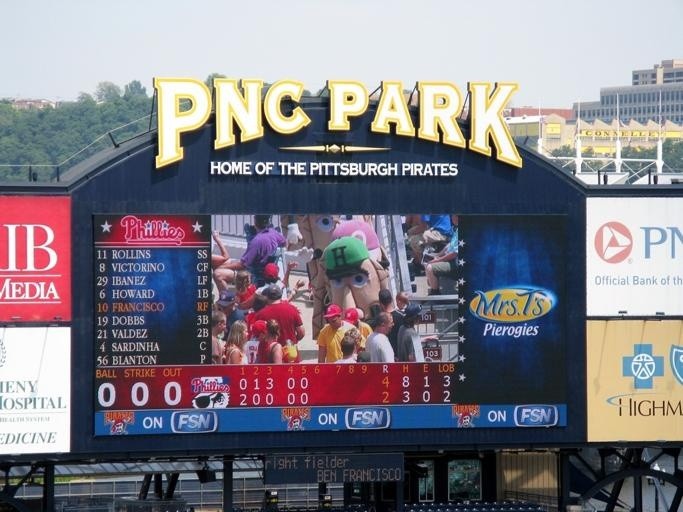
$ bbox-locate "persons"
[210,213,459,364]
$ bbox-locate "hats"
[405,304,422,319]
[216,289,236,307]
[323,304,342,319]
[342,308,358,324]
[264,262,279,278]
[261,285,281,300]
[251,320,267,337]
